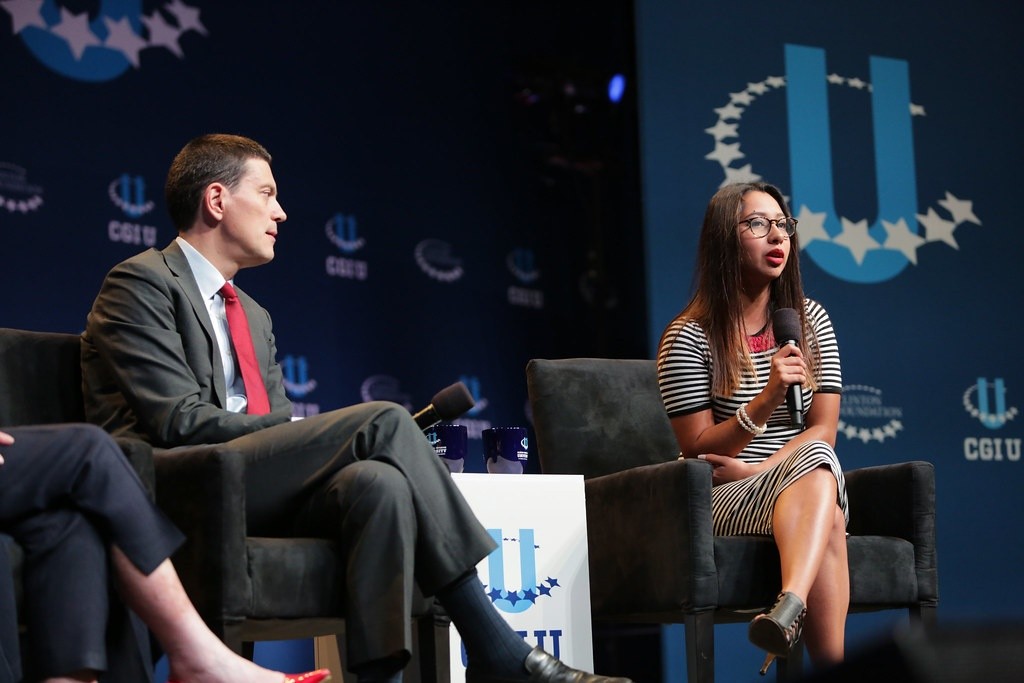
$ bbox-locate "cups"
[424,425,468,473]
[482,426,528,474]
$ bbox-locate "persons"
[655,182,851,668]
[0,423,333,683]
[83,135,632,683]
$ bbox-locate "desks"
[400,471,596,683]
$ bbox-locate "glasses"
[739,217,798,238]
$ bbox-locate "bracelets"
[737,403,767,435]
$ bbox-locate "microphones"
[413,381,475,433]
[771,308,804,426]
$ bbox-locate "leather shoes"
[462,645,633,683]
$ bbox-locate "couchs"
[526,357,941,682]
[0,326,452,682]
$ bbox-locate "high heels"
[747,591,807,674]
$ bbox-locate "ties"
[218,281,271,416]
